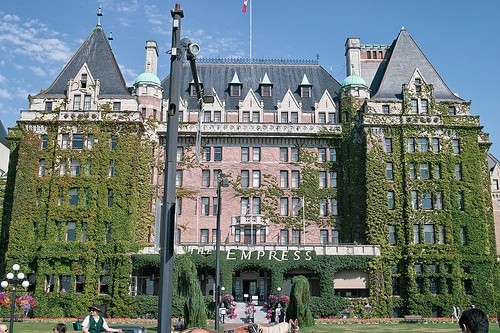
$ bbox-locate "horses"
[177,319,302,333]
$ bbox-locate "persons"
[81,304,122,333]
[459,308,489,333]
[0,323,9,333]
[52,323,67,333]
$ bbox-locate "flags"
[240,0,248,14]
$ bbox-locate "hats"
[88,304,102,312]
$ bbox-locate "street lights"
[0,264,30,333]
[156,2,206,333]
[276,287,281,302]
[221,287,225,324]
[214,178,229,332]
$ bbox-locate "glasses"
[5,328,9,332]
[89,309,96,311]
[53,328,58,331]
